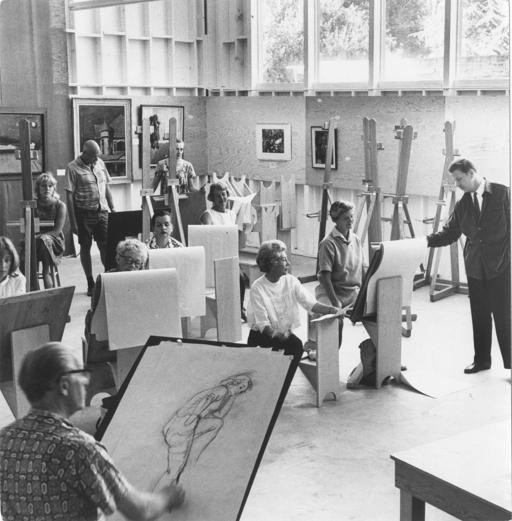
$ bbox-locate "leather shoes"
[462,363,490,374]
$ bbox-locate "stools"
[37,259,62,289]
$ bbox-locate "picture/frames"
[309,123,340,171]
[0,106,50,184]
[138,103,186,170]
[68,95,134,188]
[255,122,293,163]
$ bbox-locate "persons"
[0,234,27,299]
[144,210,184,249]
[63,139,117,297]
[103,237,148,273]
[32,171,67,288]
[245,239,339,348]
[427,159,511,374]
[0,341,186,521]
[315,199,369,309]
[150,139,198,195]
[200,181,249,321]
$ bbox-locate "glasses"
[64,368,93,379]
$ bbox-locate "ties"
[473,192,480,223]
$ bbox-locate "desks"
[385,415,512,521]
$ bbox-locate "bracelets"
[111,208,116,211]
[327,306,332,314]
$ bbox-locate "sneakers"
[87,283,95,297]
